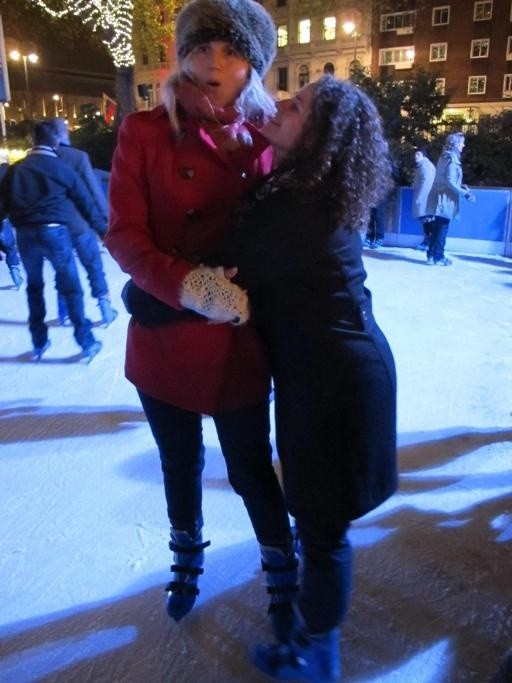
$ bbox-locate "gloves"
[179,263,249,329]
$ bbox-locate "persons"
[412,149,436,250]
[0,162,24,289]
[362,204,385,250]
[0,122,108,362]
[47,118,119,326]
[121,75,395,682]
[104,0,299,640]
[425,132,476,266]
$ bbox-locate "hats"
[176,0,278,79]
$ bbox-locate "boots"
[252,544,301,642]
[166,519,207,620]
[8,263,24,287]
[31,293,120,358]
[249,626,345,683]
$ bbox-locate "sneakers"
[362,239,383,249]
[416,240,456,265]
